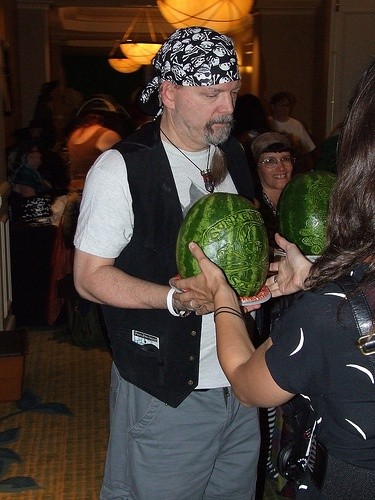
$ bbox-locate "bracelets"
[167,288,185,317]
[214,307,244,320]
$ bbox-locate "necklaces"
[159,127,215,194]
[262,193,277,215]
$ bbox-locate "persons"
[73,26,261,500]
[7,79,163,349]
[169,61,375,500]
[230,91,355,226]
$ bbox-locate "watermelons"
[275,170,339,256]
[175,192,269,298]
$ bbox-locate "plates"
[276,249,321,263]
[169,277,271,305]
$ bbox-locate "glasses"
[257,154,296,168]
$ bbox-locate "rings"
[274,276,278,283]
[190,301,194,308]
